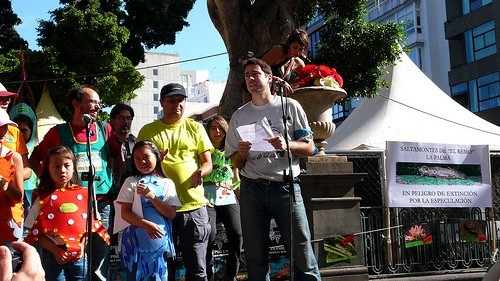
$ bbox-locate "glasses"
[116,116,133,121]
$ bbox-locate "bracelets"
[275,77,285,86]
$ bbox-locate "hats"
[160,83,186,98]
[0,106,18,128]
[0,82,18,100]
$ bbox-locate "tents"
[324,39,500,273]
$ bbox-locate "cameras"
[0,242,23,273]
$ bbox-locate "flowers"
[287,64,344,90]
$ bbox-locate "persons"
[200,116,243,281]
[109,103,137,201]
[0,83,47,280]
[29,86,122,281]
[242,29,310,105]
[116,140,182,281]
[224,58,322,281]
[136,83,210,281]
[24,146,110,280]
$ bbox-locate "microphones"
[273,80,285,89]
[82,114,92,124]
